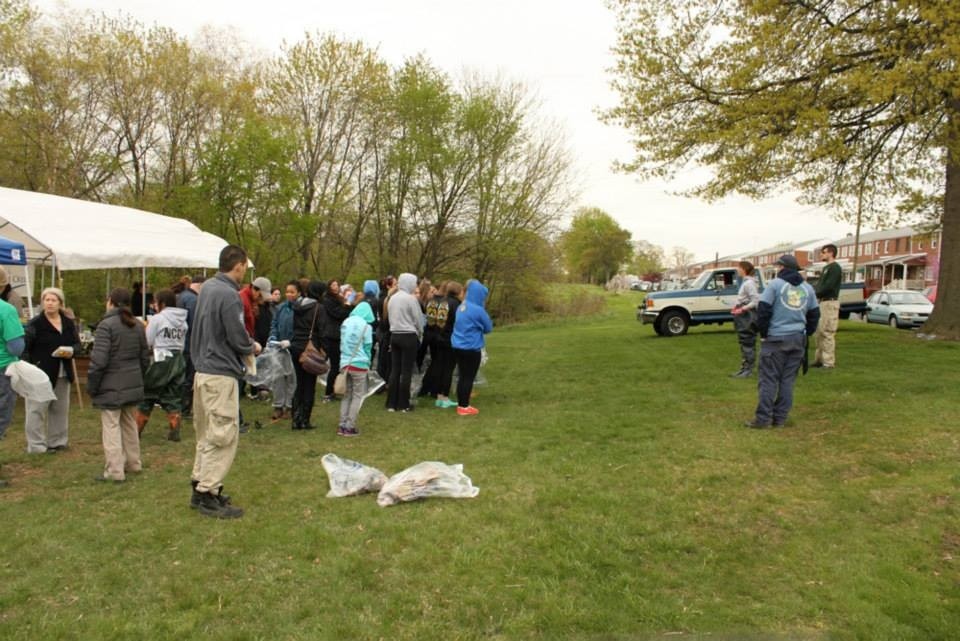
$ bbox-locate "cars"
[922,285,938,304]
[864,289,935,330]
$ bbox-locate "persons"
[0,268,22,437]
[190,244,262,520]
[809,244,842,371]
[743,254,820,428]
[137,288,189,443]
[337,299,373,435]
[131,273,493,431]
[25,287,81,455]
[728,260,759,378]
[86,286,148,484]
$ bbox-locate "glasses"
[822,250,831,254]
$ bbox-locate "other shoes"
[1,386,479,457]
[817,366,833,371]
[95,473,126,482]
[745,420,773,430]
[123,462,142,473]
[808,362,823,369]
[773,423,783,429]
[728,369,752,378]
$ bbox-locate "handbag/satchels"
[300,341,329,375]
[334,374,347,395]
[5,360,58,402]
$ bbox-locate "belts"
[819,297,837,301]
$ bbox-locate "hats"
[0,268,10,287]
[252,276,273,300]
[41,287,65,311]
[774,254,798,268]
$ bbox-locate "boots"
[199,485,244,519]
[190,481,232,511]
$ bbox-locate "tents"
[0,186,255,412]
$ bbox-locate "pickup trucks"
[635,264,873,339]
